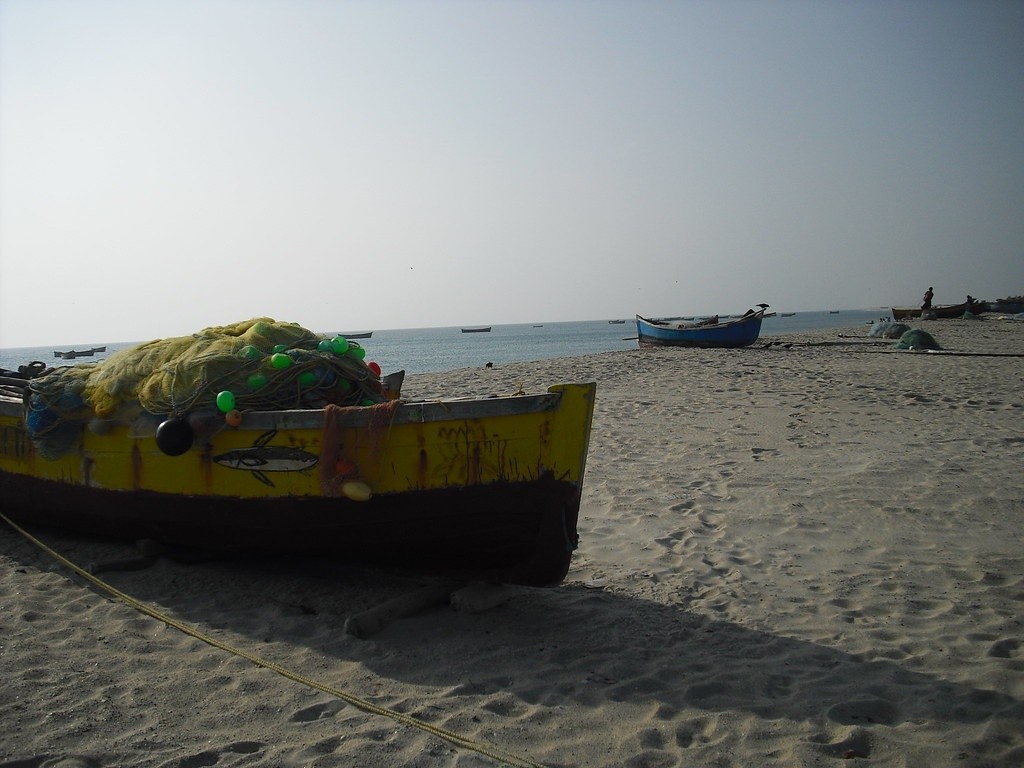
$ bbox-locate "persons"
[869,315,891,324]
[967,295,971,302]
[922,287,934,314]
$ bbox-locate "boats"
[635,303,770,348]
[461,326,491,333]
[0,367,599,586]
[338,331,373,339]
[608,320,625,324]
[892,295,1024,320]
[54,346,106,360]
